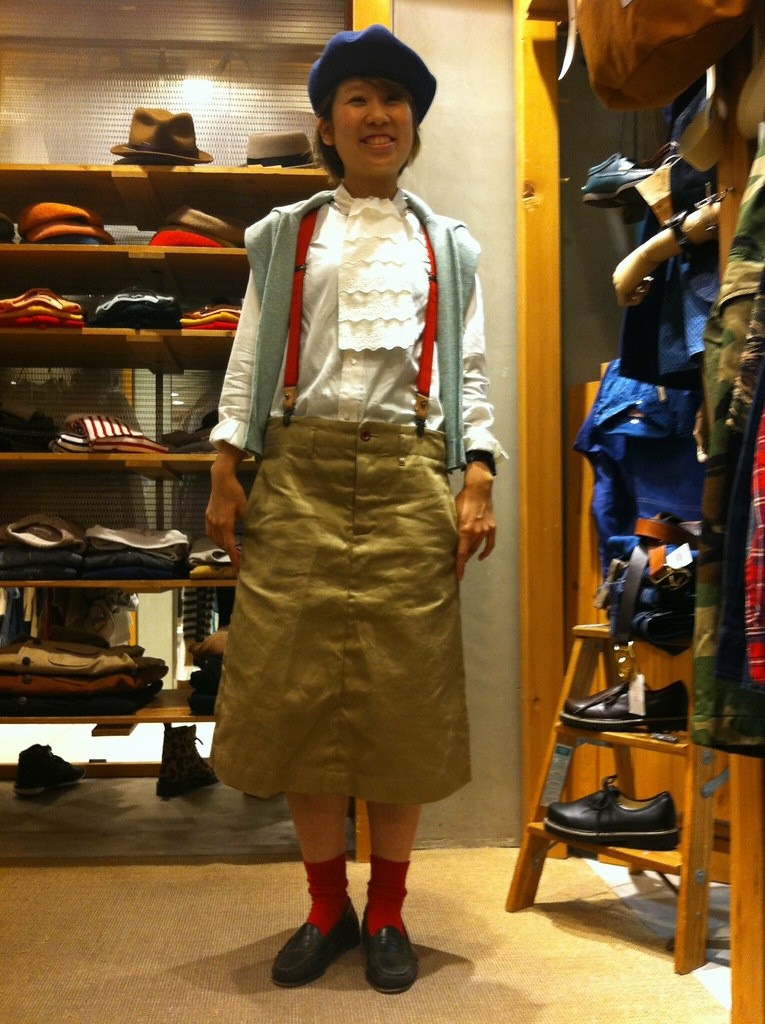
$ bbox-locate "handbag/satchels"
[576,0,753,110]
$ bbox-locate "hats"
[238,130,319,168]
[109,107,214,163]
[306,24,436,125]
[0,203,249,251]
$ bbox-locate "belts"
[594,514,703,663]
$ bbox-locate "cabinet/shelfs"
[2,161,369,867]
[508,1,765,1023]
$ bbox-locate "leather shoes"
[558,673,688,733]
[542,775,679,849]
[271,896,361,987]
[360,903,418,994]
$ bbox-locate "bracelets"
[666,209,690,249]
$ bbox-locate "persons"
[205,24,507,994]
[614,56,765,757]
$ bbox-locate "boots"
[156,722,218,796]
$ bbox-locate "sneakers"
[582,153,659,209]
[15,745,86,796]
